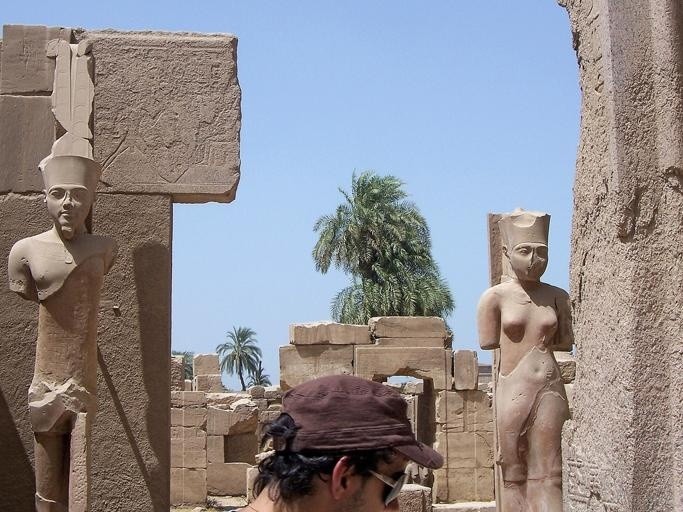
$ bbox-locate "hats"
[272,376,444,469]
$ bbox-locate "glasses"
[352,458,407,505]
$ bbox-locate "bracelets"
[249,505,258,512]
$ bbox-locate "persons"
[8,153,116,512]
[230,374,443,512]
[477,208,574,512]
